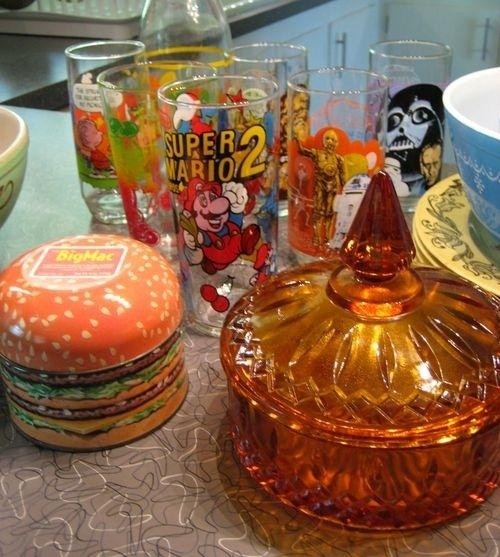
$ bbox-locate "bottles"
[215,169,499,532]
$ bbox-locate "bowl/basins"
[437,62,499,241]
[0,105,28,225]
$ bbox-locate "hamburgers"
[0,233,190,452]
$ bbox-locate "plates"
[405,172,499,311]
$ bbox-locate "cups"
[96,60,217,250]
[139,0,232,77]
[365,39,454,213]
[223,42,306,218]
[283,67,389,268]
[63,40,146,227]
[158,74,282,337]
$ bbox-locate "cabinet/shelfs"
[380,0,500,96]
[275,0,380,142]
